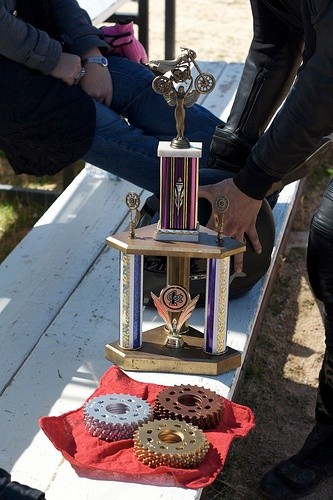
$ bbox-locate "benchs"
[0,61,306,500]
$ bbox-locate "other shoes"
[261,456,327,500]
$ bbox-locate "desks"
[75,0,175,62]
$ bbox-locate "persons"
[170,75,194,140]
[197,0,333,500]
[0,0,333,209]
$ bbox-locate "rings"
[79,67,85,76]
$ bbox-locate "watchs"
[81,56,108,66]
